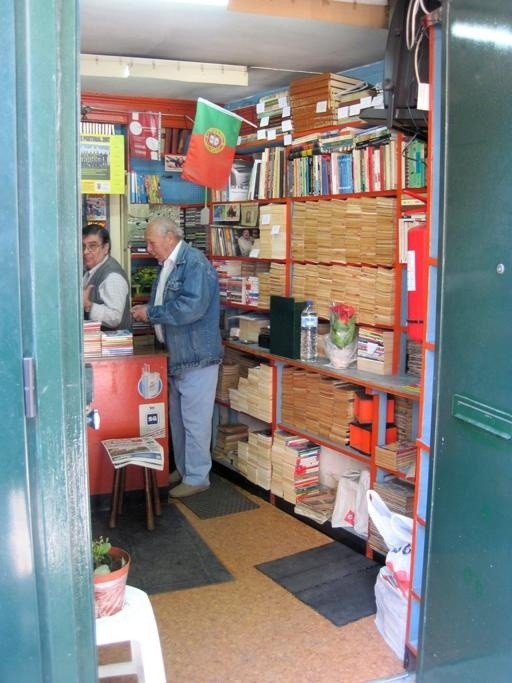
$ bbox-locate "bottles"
[298,299,317,360]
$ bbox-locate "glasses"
[83,243,104,251]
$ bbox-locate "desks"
[83,348,170,495]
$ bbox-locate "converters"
[417,82,429,111]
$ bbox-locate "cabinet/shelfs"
[80,94,222,339]
[221,58,428,562]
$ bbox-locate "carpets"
[256,541,382,629]
[178,471,261,519]
[91,489,236,594]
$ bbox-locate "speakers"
[269,294,308,359]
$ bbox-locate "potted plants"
[91,533,132,620]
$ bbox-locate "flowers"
[327,300,356,346]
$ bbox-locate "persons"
[82,224,133,333]
[131,217,225,497]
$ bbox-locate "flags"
[181,101,243,191]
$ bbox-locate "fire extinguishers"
[403,189,428,343]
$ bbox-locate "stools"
[107,440,162,530]
[95,587,166,682]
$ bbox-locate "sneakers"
[168,470,181,482]
[168,483,209,498]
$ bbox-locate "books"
[209,344,274,491]
[270,365,418,556]
[210,73,427,202]
[79,112,208,255]
[290,195,427,379]
[211,260,286,348]
[83,265,155,359]
[210,202,287,259]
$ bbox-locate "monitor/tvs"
[383,0,442,107]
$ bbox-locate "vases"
[332,347,353,369]
